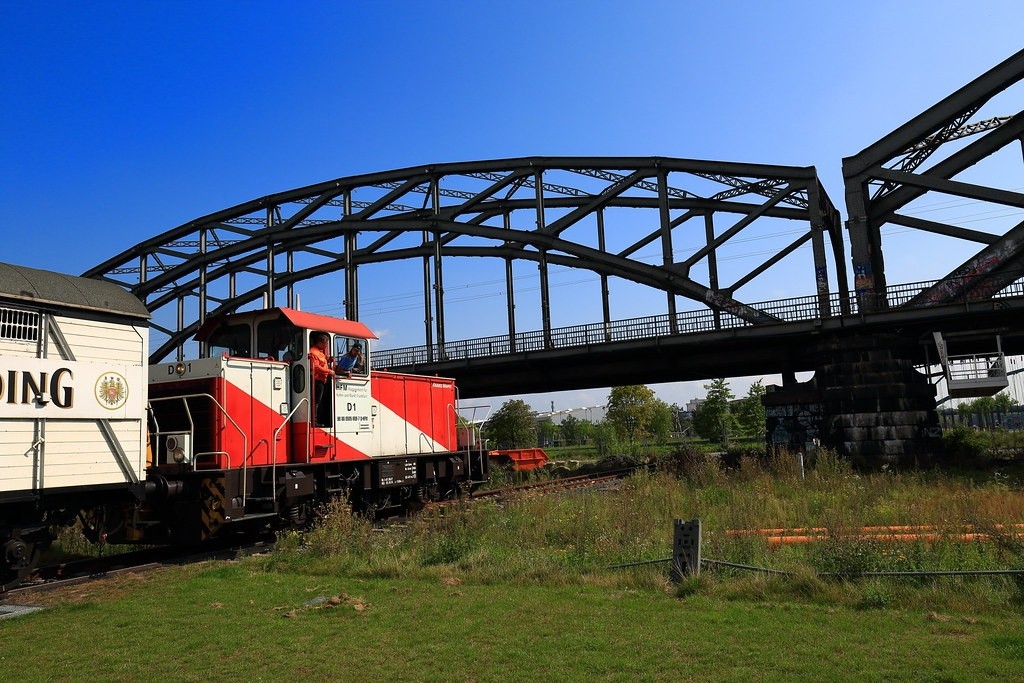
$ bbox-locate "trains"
[0,261,492,597]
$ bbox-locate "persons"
[335,343,365,377]
[310,335,336,427]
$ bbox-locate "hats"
[353,343,362,351]
